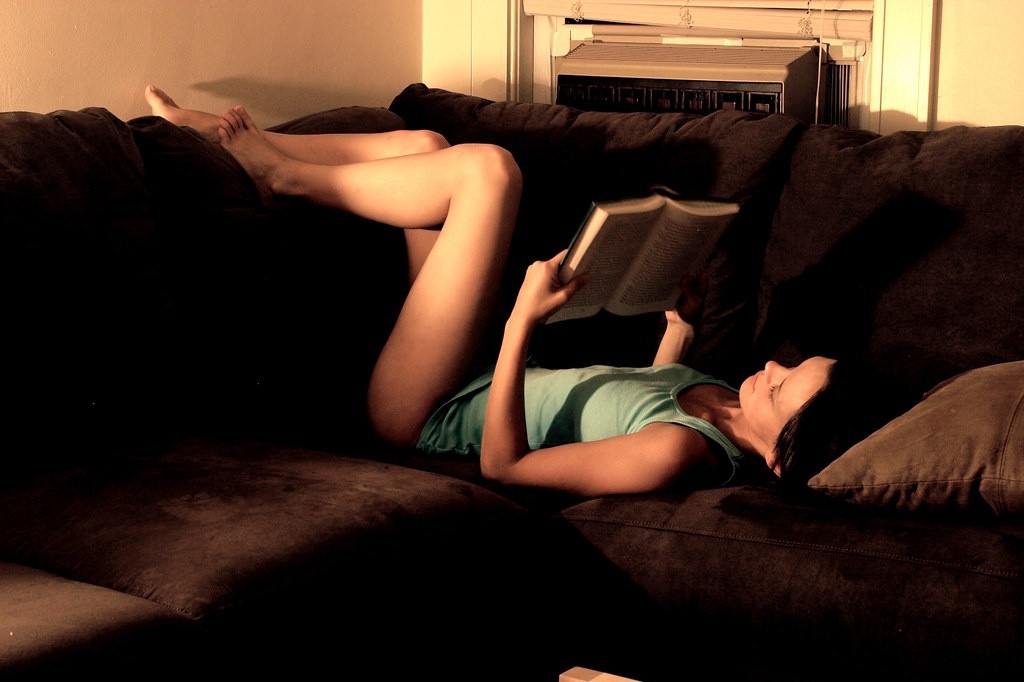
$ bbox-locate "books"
[536,182,748,327]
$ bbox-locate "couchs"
[0,81,1024,682]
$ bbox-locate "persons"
[145,86,917,498]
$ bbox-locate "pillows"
[807,361,1024,515]
